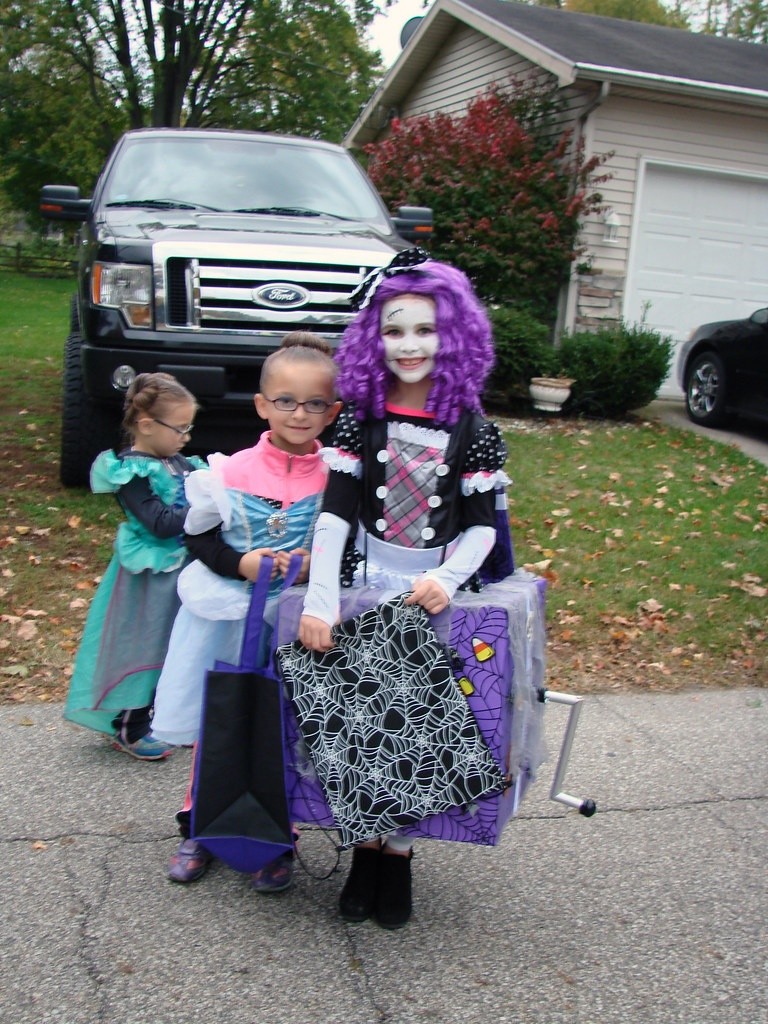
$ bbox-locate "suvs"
[36,128,440,493]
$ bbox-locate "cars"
[677,305,768,434]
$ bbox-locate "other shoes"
[340,843,413,928]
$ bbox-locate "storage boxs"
[276,569,549,839]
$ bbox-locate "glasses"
[135,419,194,434]
[264,398,333,413]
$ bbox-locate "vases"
[529,377,572,412]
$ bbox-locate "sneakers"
[253,849,293,892]
[169,838,206,882]
[111,728,172,760]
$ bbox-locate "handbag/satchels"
[190,555,304,871]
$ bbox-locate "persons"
[151,331,342,892]
[62,365,209,760]
[298,247,515,929]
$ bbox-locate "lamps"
[603,207,622,243]
